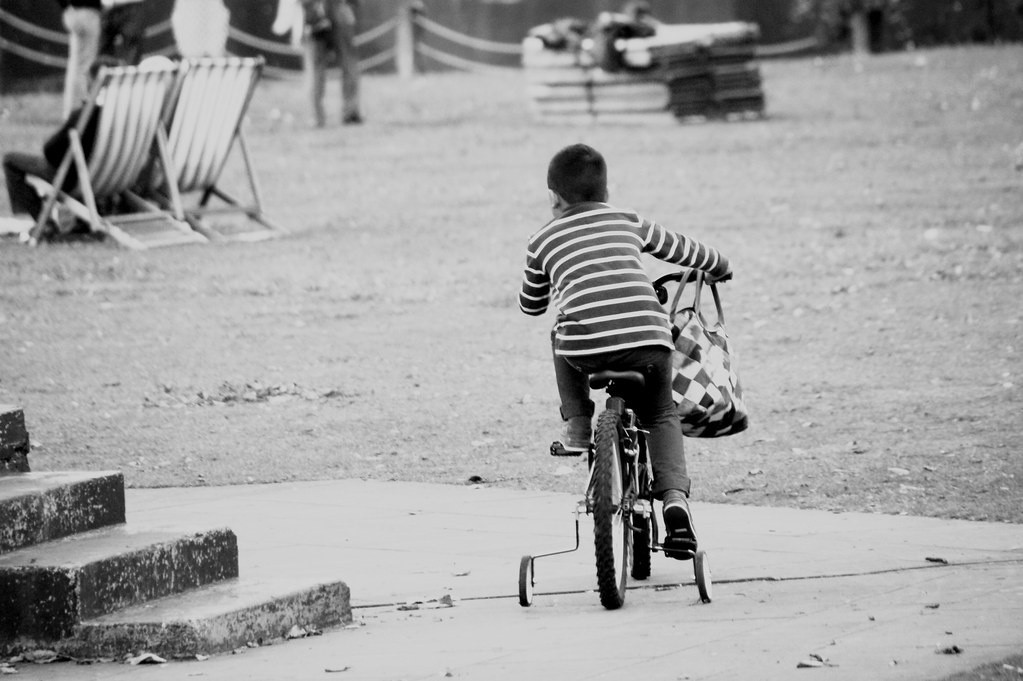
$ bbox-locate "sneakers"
[556,417,592,452]
[663,490,697,560]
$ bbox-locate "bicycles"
[516,262,738,609]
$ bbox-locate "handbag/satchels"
[667,268,749,438]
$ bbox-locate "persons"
[301,1,365,129]
[517,142,734,560]
[58,0,106,127]
[2,54,131,241]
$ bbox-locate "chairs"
[130,55,293,246]
[25,59,210,255]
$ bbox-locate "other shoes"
[19,215,89,242]
